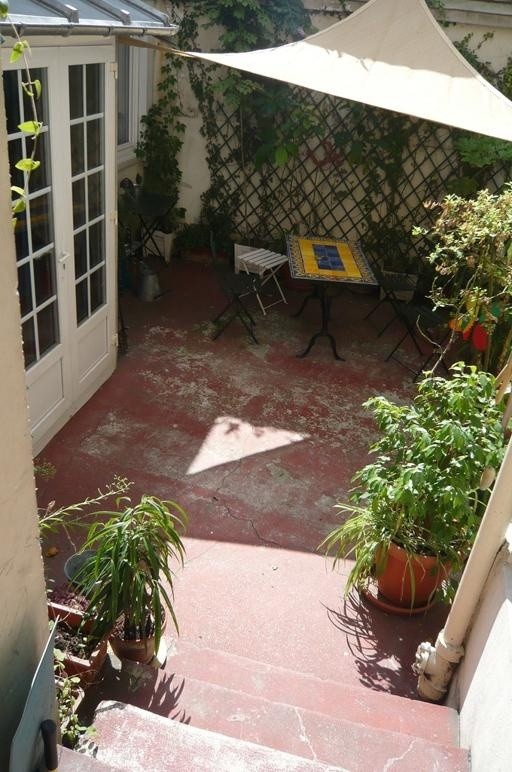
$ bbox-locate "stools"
[237,247,289,315]
[213,273,258,345]
[385,303,450,383]
[364,270,422,343]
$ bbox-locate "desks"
[118,191,178,269]
[283,234,383,361]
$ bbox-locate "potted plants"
[70,494,192,668]
[318,357,504,616]
